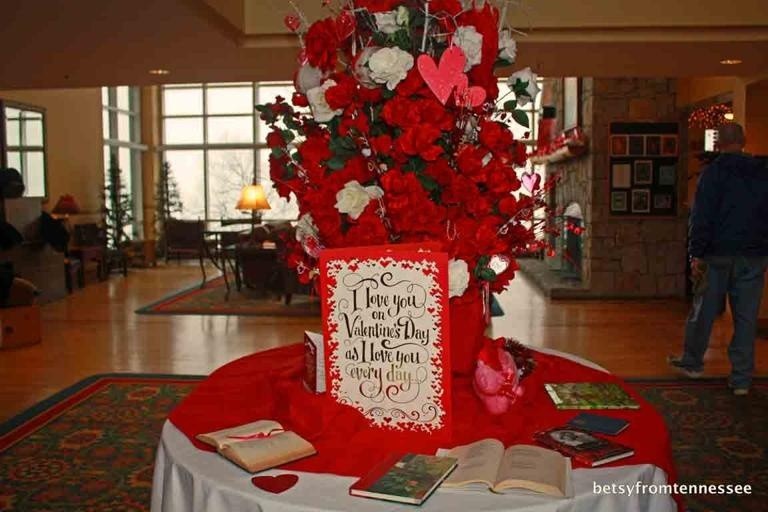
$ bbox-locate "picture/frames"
[560,76,581,132]
[608,133,678,216]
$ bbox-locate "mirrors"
[1,99,49,203]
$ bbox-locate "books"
[546,380,640,410]
[567,411,630,436]
[536,421,634,467]
[436,438,576,498]
[348,448,459,506]
[196,417,319,474]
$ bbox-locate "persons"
[667,122,768,395]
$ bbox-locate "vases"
[448,283,484,377]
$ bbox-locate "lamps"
[235,186,273,249]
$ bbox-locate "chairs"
[161,218,207,260]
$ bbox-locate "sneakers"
[668,356,702,380]
[727,375,750,395]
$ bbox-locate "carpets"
[0,375,768,512]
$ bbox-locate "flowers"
[254,1,582,296]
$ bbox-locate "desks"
[161,336,673,512]
[200,223,251,296]
